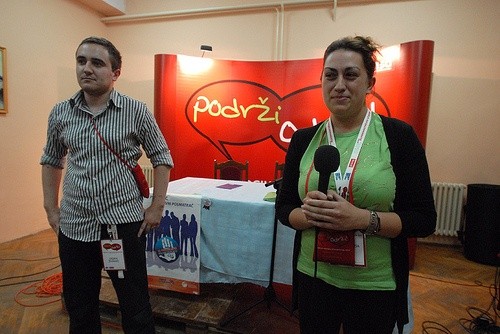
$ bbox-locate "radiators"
[430,181,466,240]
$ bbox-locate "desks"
[139,173,298,304]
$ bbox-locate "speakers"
[463,183,500,268]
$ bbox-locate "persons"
[278,34,438,334]
[38,35,174,334]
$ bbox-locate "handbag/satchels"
[76,98,150,198]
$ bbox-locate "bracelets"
[361,210,381,236]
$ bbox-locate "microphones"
[313,146,341,235]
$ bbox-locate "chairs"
[213,158,249,181]
[275,162,284,179]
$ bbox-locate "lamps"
[199,44,212,57]
